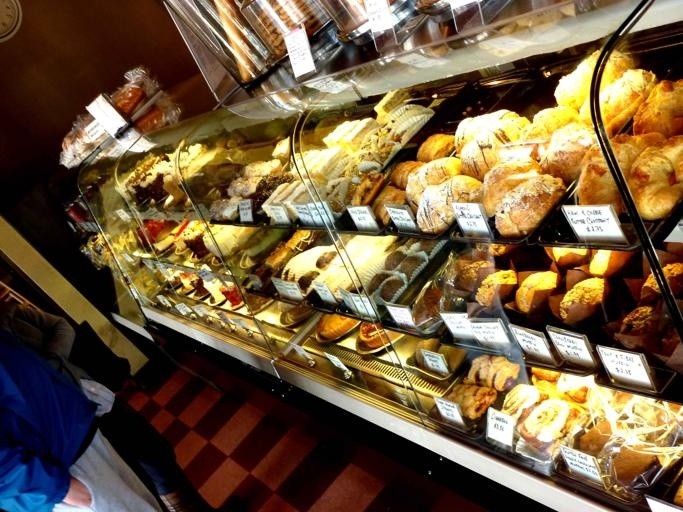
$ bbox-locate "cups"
[219,282,243,306]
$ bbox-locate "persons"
[0,330,165,512]
[0,280,249,511]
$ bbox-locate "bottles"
[65,204,89,223]
[164,0,417,92]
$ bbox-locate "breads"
[61,50,682,507]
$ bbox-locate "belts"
[66,415,99,468]
